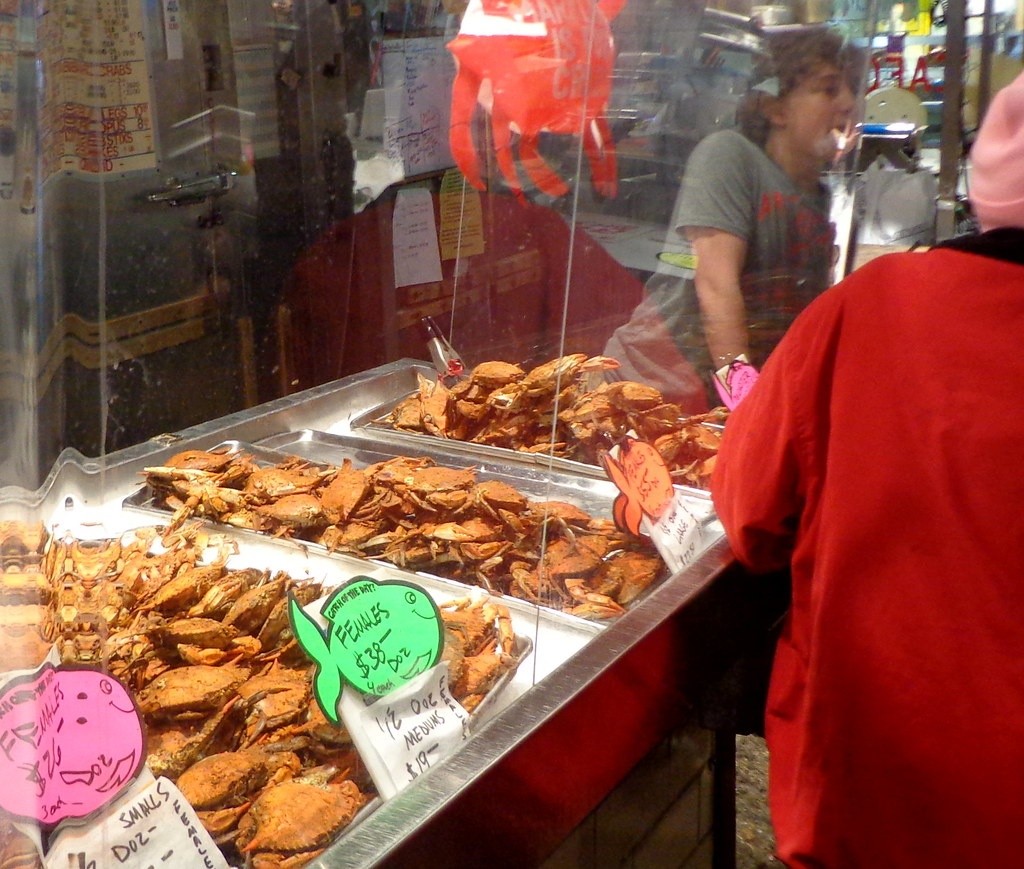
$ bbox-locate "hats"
[970,72,1024,233]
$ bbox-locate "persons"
[583,21,866,410]
[709,70,1024,869]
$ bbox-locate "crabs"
[0,352,733,869]
